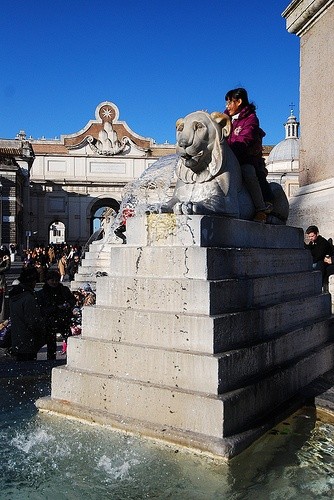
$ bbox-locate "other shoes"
[252,212,266,223]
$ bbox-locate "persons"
[0,241,96,362]
[304,226,334,286]
[114,210,134,244]
[219,88,274,222]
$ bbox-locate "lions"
[114,110,292,225]
